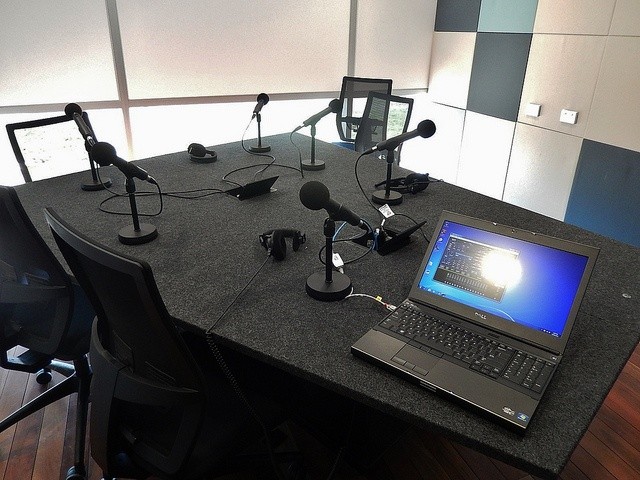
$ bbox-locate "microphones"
[374,118,444,154]
[92,141,159,189]
[299,97,343,130]
[298,180,373,238]
[246,90,272,123]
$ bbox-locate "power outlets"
[560,109,578,125]
[525,103,541,117]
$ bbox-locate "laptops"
[349,205,599,432]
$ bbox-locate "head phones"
[181,140,226,168]
[377,169,441,198]
[252,222,305,257]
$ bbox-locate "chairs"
[43,204,299,479]
[355,89,414,167]
[336,75,392,143]
[1,186,100,479]
[5,112,98,183]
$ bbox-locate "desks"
[7,131,638,479]
